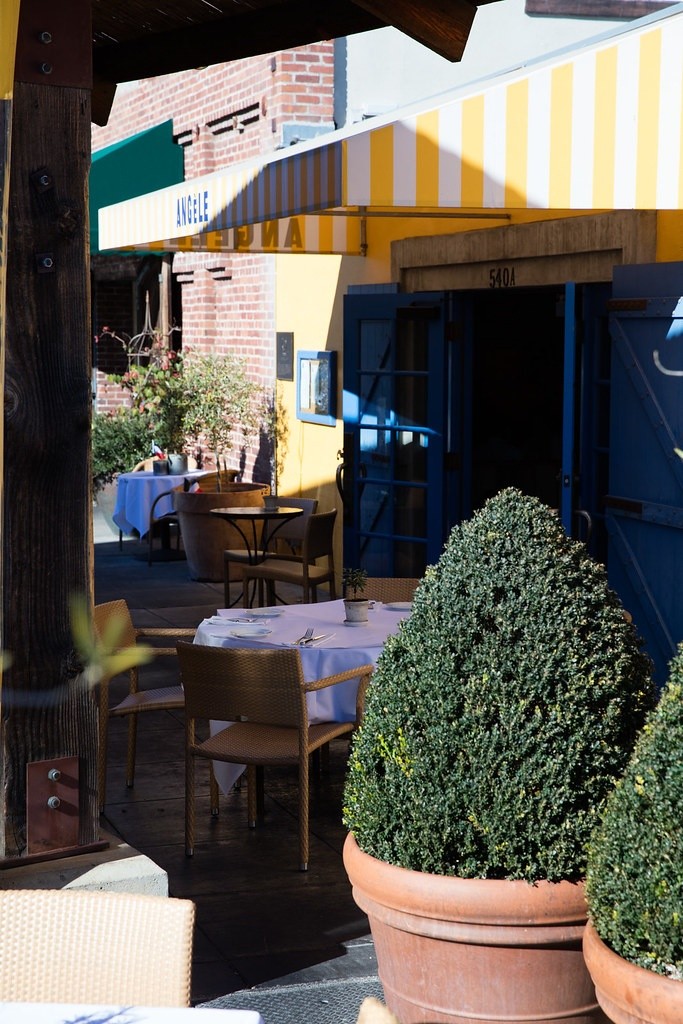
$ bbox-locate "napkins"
[205,616,271,624]
[281,632,337,647]
[374,601,382,610]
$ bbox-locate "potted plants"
[342,568,369,622]
[262,492,280,511]
[173,348,285,582]
[146,378,191,473]
[342,486,683,1024]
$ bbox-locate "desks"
[115,469,216,551]
[209,506,304,609]
[182,599,415,823]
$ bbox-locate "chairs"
[133,453,240,567]
[243,496,337,609]
[93,577,421,871]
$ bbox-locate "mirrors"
[297,349,338,426]
